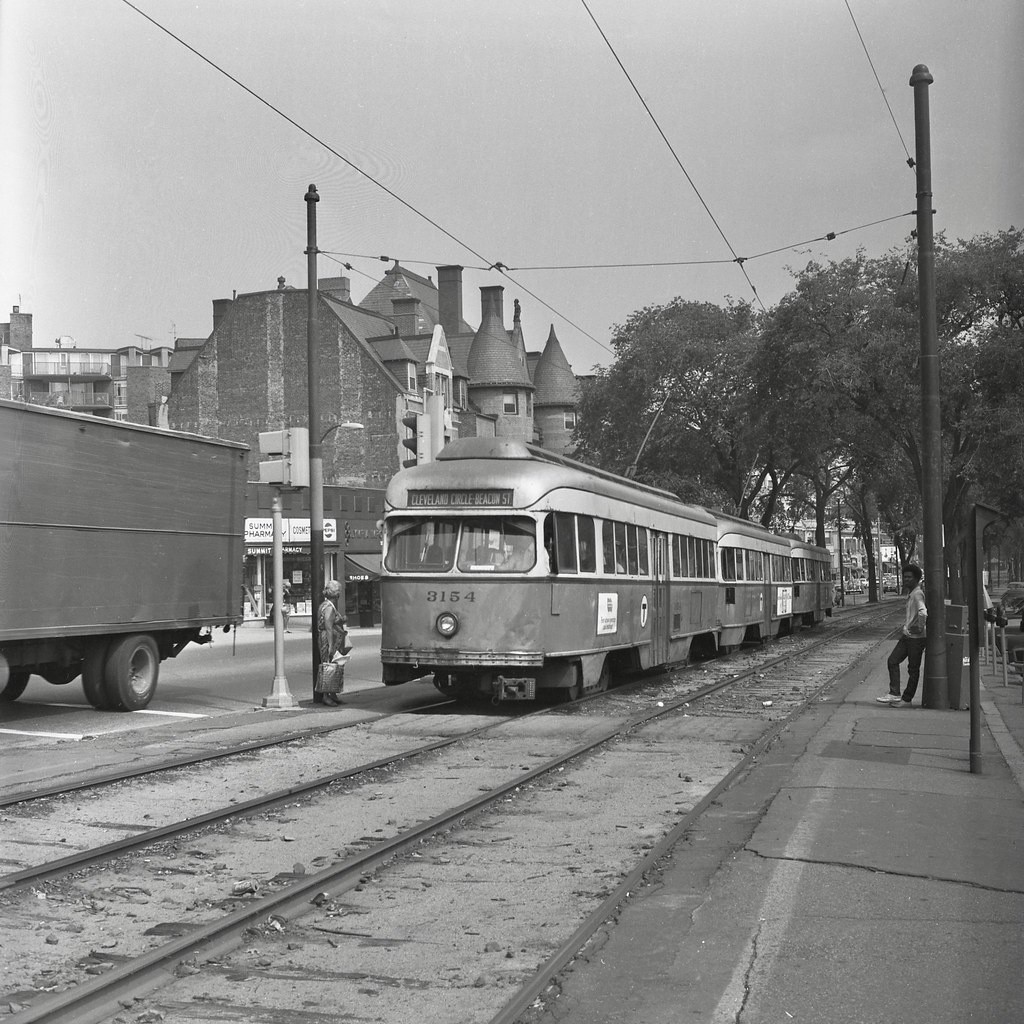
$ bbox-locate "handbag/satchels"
[315,654,344,693]
[339,632,353,655]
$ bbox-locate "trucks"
[0,398,248,717]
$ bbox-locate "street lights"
[307,422,367,705]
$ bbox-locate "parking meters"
[985,606,998,674]
[996,605,1009,687]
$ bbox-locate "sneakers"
[876,693,901,703]
[889,698,912,708]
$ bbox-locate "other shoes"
[324,695,344,707]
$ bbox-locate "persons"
[876,564,928,708]
[318,580,345,707]
[283,582,292,633]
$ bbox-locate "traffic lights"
[258,425,308,493]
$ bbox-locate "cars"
[842,572,901,596]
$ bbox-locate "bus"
[376,433,839,707]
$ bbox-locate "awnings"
[344,554,382,583]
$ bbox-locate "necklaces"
[327,600,336,612]
